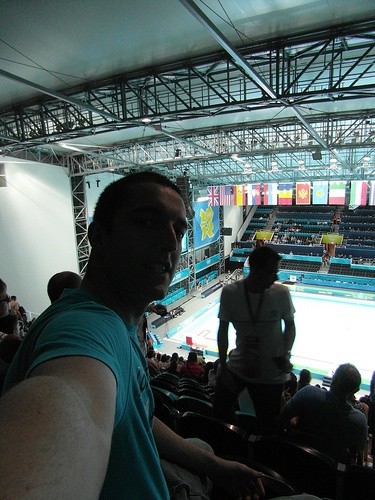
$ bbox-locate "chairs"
[149,361,375,500]
[230,204,375,278]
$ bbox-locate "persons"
[0,271,82,378]
[274,364,369,467]
[286,369,375,448]
[212,246,295,437]
[144,347,221,389]
[0,170,267,500]
